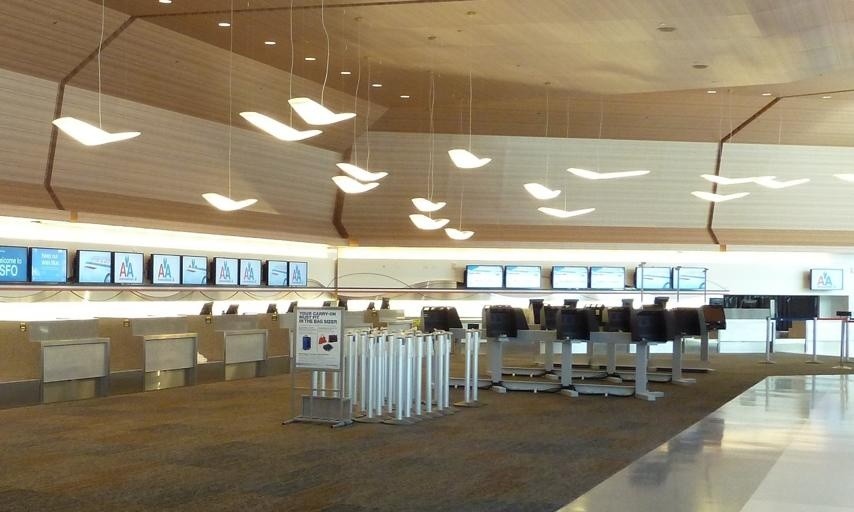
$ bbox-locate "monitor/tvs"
[28,247,67,283]
[147,254,181,285]
[552,266,625,290]
[262,260,288,286]
[74,250,112,282]
[287,261,308,287]
[111,252,144,285]
[180,255,208,285]
[466,264,541,288]
[635,267,706,289]
[200,300,297,315]
[810,268,844,290]
[529,295,668,311]
[324,297,390,310]
[210,258,239,286]
[239,259,261,285]
[0,245,29,281]
[709,298,725,307]
